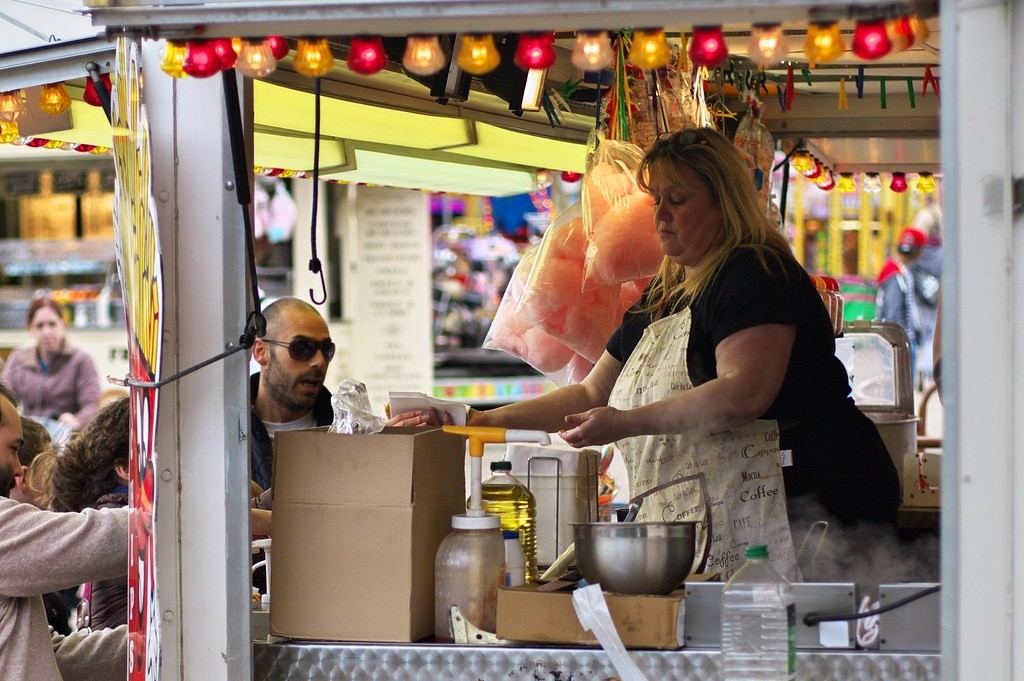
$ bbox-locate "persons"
[425,127,900,584]
[0,296,428,681]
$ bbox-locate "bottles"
[500,530,526,587]
[466,461,539,585]
[720,543,798,681]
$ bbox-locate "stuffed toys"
[485,162,665,385]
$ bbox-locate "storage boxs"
[496,581,687,650]
[268,425,467,644]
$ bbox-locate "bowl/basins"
[565,521,695,595]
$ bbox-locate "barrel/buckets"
[859,412,922,502]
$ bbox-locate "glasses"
[260,336,336,363]
